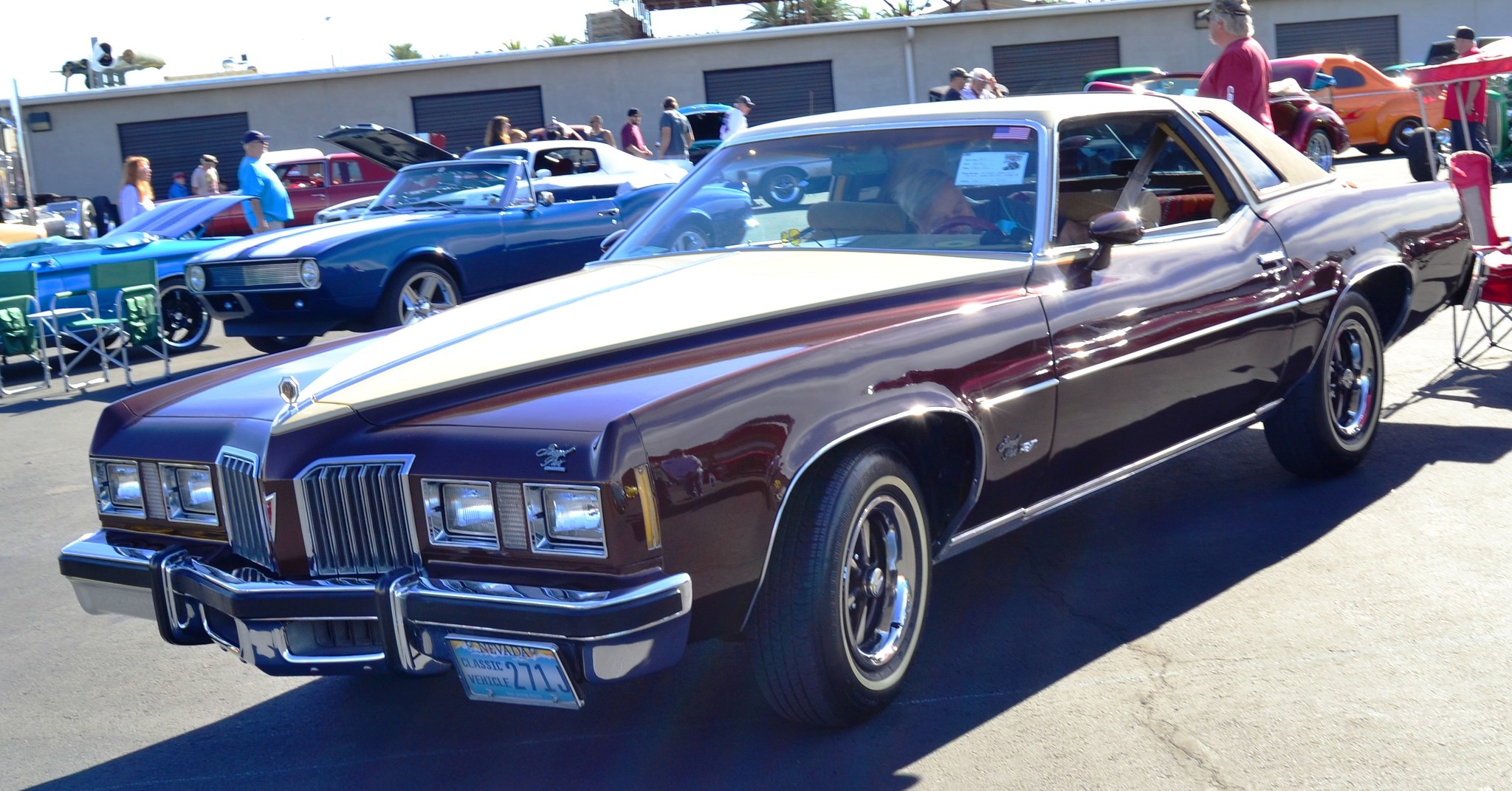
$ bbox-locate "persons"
[1444,27,1508,185]
[1199,0,1275,132]
[238,131,295,234]
[718,96,755,141]
[169,153,229,198]
[898,168,1092,248]
[943,67,1000,100]
[117,156,155,224]
[483,115,615,149]
[659,97,695,159]
[621,109,653,159]
[663,448,705,499]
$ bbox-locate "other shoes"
[1496,169,1508,181]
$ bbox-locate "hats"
[240,130,271,145]
[628,108,645,117]
[734,96,756,107]
[1194,9,1247,29]
[1447,26,1474,40]
[202,154,219,163]
[950,67,973,81]
[173,172,189,178]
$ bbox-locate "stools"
[25,306,107,395]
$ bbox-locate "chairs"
[45,258,170,391]
[0,258,50,397]
[1441,150,1512,369]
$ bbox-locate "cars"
[1068,29,1512,184]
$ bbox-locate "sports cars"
[58,91,1486,719]
[0,103,838,354]
